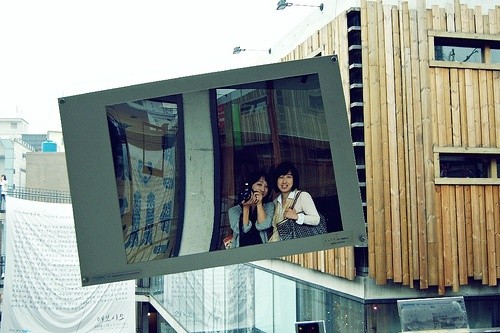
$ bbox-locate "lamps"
[276,0,323,11]
[233,47,272,55]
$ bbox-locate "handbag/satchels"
[222,234,233,250]
[276,190,328,241]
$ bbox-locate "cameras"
[239,183,254,203]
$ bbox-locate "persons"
[228,161,326,248]
